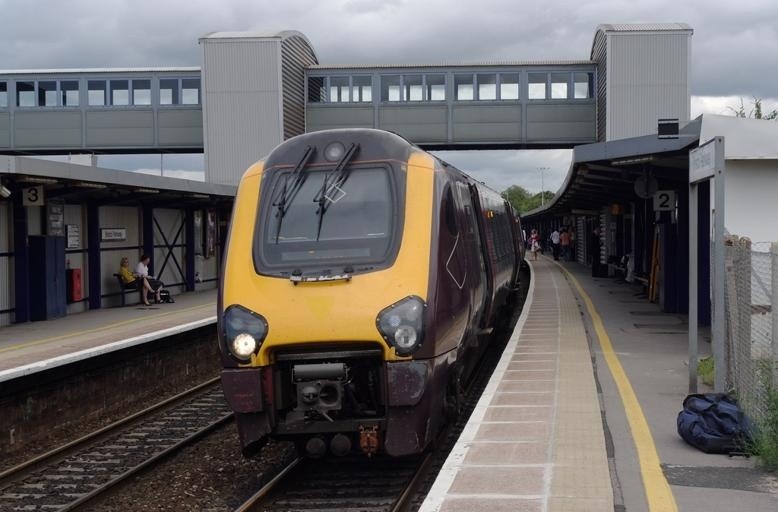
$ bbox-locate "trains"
[216,127,526,460]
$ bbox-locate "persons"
[521,226,572,262]
[120,257,159,306]
[134,254,166,304]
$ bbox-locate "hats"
[532,228,536,232]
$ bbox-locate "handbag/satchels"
[677,392,750,454]
[535,235,541,240]
[527,238,531,244]
[155,290,169,303]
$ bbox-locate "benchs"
[113,272,154,306]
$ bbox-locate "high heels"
[142,300,152,306]
[151,288,155,293]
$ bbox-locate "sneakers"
[156,300,164,304]
